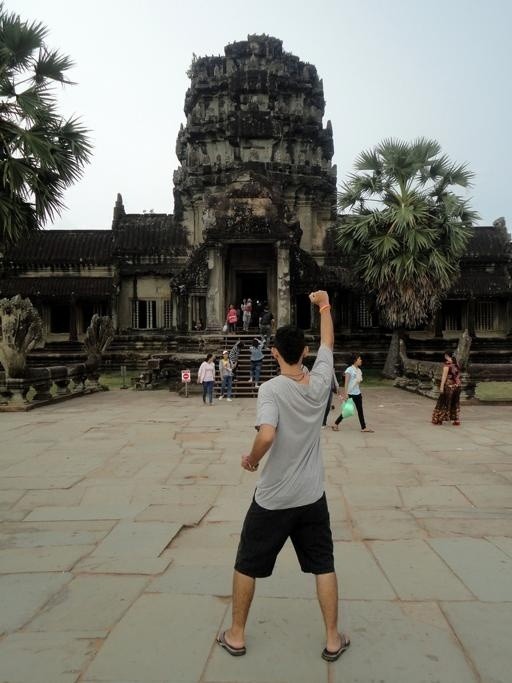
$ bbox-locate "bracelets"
[245,455,260,471]
[319,303,332,312]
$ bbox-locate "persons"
[429,350,464,427]
[218,297,274,402]
[196,354,216,404]
[330,352,373,434]
[321,367,339,428]
[215,290,352,661]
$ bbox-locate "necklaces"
[276,369,310,383]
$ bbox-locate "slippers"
[323,634,350,660]
[216,630,245,655]
